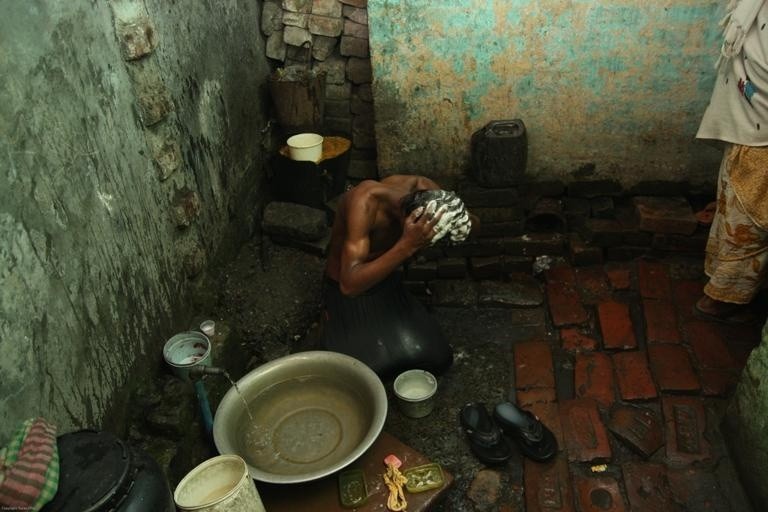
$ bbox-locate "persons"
[694,0,768,325]
[316,175,470,379]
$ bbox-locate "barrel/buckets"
[175,455,266,512]
[162,330,212,383]
[266,42,327,132]
[35,429,174,511]
[393,370,438,419]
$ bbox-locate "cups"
[200,320,215,335]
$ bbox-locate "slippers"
[691,302,766,330]
[461,403,512,464]
[494,401,558,463]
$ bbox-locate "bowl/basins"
[212,350,390,483]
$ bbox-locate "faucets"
[190,364,225,377]
